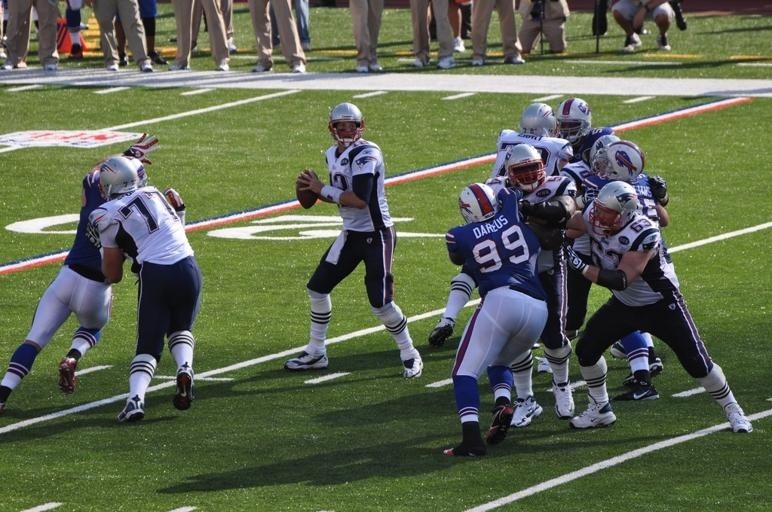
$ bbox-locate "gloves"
[648,175,668,202]
[165,188,185,212]
[124,132,158,164]
[561,240,585,271]
[85,223,102,250]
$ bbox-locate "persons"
[350,1,383,73]
[1,0,169,73]
[611,1,675,53]
[1,132,187,416]
[280,100,428,380]
[426,98,756,459]
[410,0,525,69]
[168,0,312,73]
[519,0,570,57]
[83,157,202,423]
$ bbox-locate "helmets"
[504,144,545,192]
[459,182,500,223]
[328,103,365,142]
[590,135,644,181]
[121,156,148,183]
[99,157,139,194]
[557,98,592,145]
[521,103,559,136]
[590,181,638,235]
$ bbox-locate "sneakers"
[106,48,197,72]
[370,63,382,72]
[252,63,272,72]
[429,317,455,346]
[411,36,525,69]
[45,62,58,70]
[283,350,329,370]
[610,340,664,402]
[403,353,424,378]
[116,395,145,421]
[228,36,237,55]
[292,60,306,72]
[724,401,753,433]
[624,36,642,52]
[656,34,671,50]
[68,43,83,59]
[174,362,194,411]
[1,61,14,70]
[356,64,368,73]
[553,379,575,419]
[569,393,616,429]
[217,63,230,71]
[443,394,543,456]
[58,356,76,394]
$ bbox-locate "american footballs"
[296,169,319,208]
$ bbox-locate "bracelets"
[320,184,343,204]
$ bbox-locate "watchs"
[644,4,649,12]
